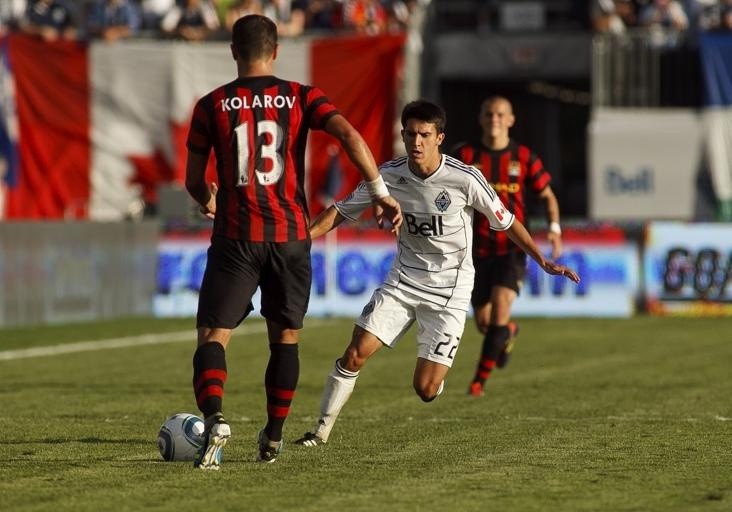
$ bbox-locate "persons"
[185,14,400,473]
[686,2,731,223]
[295,99,584,448]
[450,94,565,397]
[0,1,732,108]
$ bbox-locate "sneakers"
[495,321,519,369]
[256,429,282,465]
[196,411,231,470]
[294,431,325,447]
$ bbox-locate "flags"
[0,32,402,246]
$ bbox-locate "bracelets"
[361,174,390,204]
[549,223,564,236]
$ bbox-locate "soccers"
[157,413,206,460]
